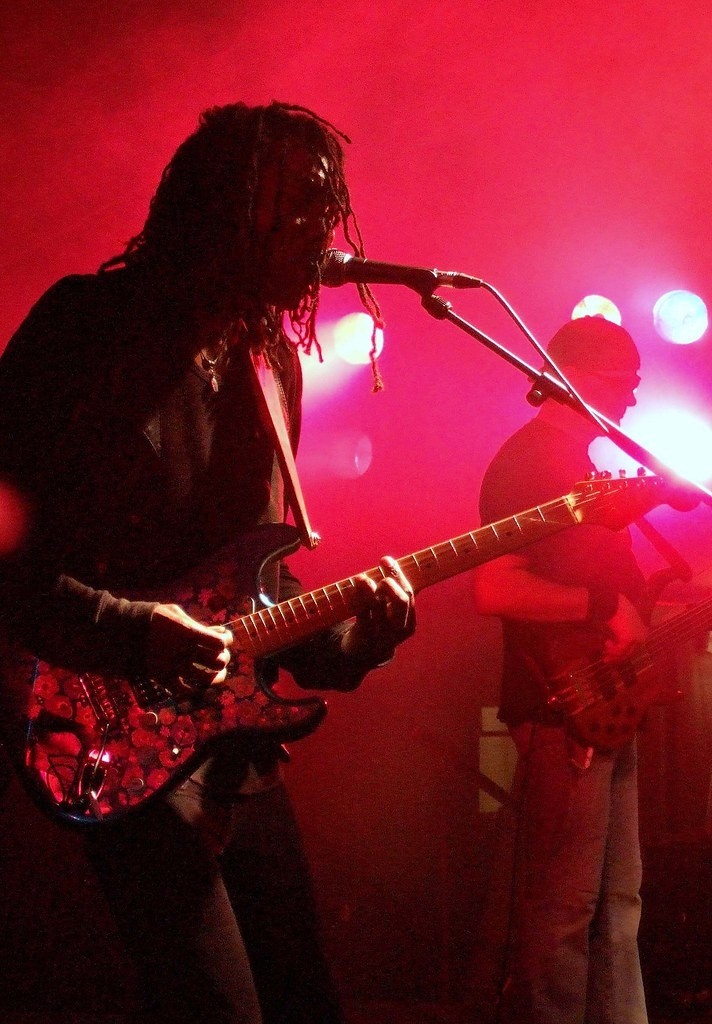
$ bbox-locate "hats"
[527,313,641,407]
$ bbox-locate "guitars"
[0,466,702,832]
[529,559,711,754]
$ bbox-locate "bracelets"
[335,628,396,670]
[587,583,619,623]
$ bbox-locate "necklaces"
[190,329,229,393]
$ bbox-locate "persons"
[479,315,712,1024]
[0,100,415,1024]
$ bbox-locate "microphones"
[318,248,482,289]
